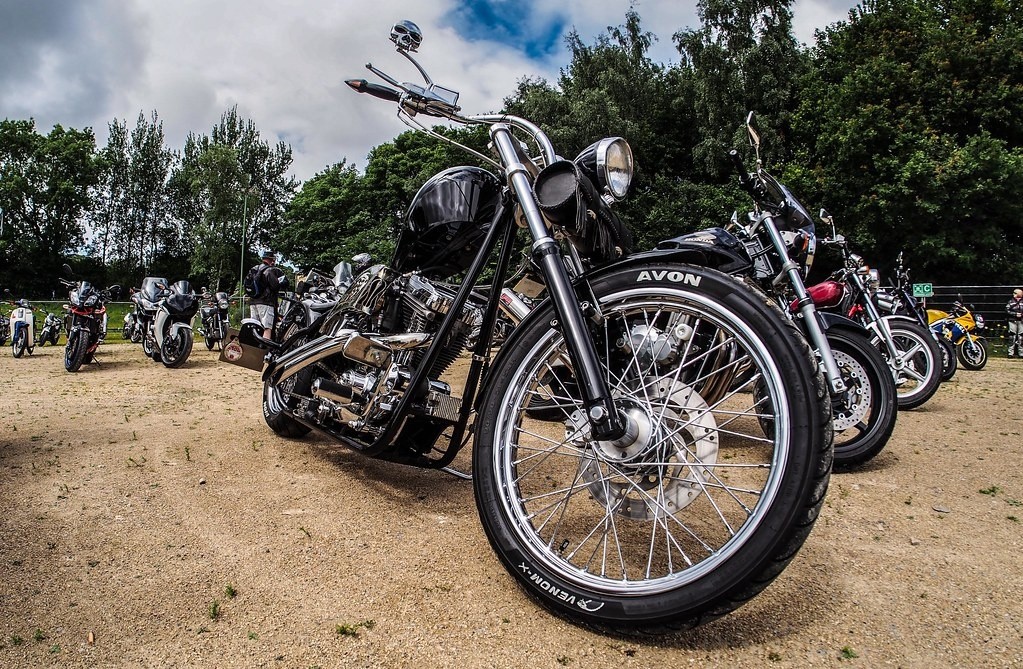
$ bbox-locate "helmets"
[260,250,274,258]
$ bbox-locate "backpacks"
[244,266,270,298]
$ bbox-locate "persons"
[244,251,290,339]
[1006,289,1023,358]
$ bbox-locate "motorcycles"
[123,276,240,367]
[237,19,989,639]
[0,264,122,372]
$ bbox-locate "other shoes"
[1008,355,1014,358]
